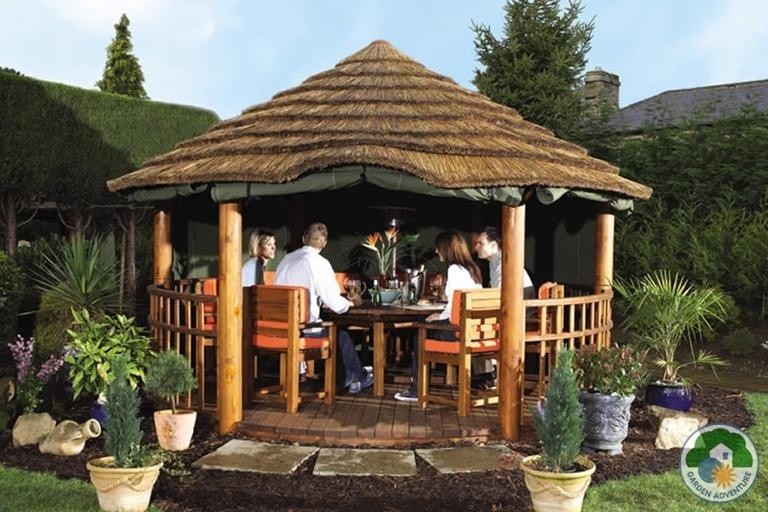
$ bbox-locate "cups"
[347,279,367,300]
[388,272,446,306]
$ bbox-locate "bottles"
[38,418,102,456]
[371,279,380,308]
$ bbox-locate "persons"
[393,229,486,401]
[470,225,537,392]
[273,222,376,395]
[241,228,277,286]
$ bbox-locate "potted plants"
[518,343,597,512]
[606,269,739,411]
[61,307,155,448]
[575,342,645,455]
[86,355,166,512]
[143,348,198,454]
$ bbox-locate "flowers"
[339,225,439,274]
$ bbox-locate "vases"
[378,272,391,289]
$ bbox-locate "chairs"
[524,279,566,406]
[263,270,279,283]
[417,287,502,416]
[241,284,337,415]
[192,274,261,382]
[329,270,394,368]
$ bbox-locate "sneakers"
[349,373,374,393]
[471,366,497,390]
[394,391,419,402]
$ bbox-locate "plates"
[404,302,445,311]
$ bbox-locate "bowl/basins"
[368,288,403,306]
